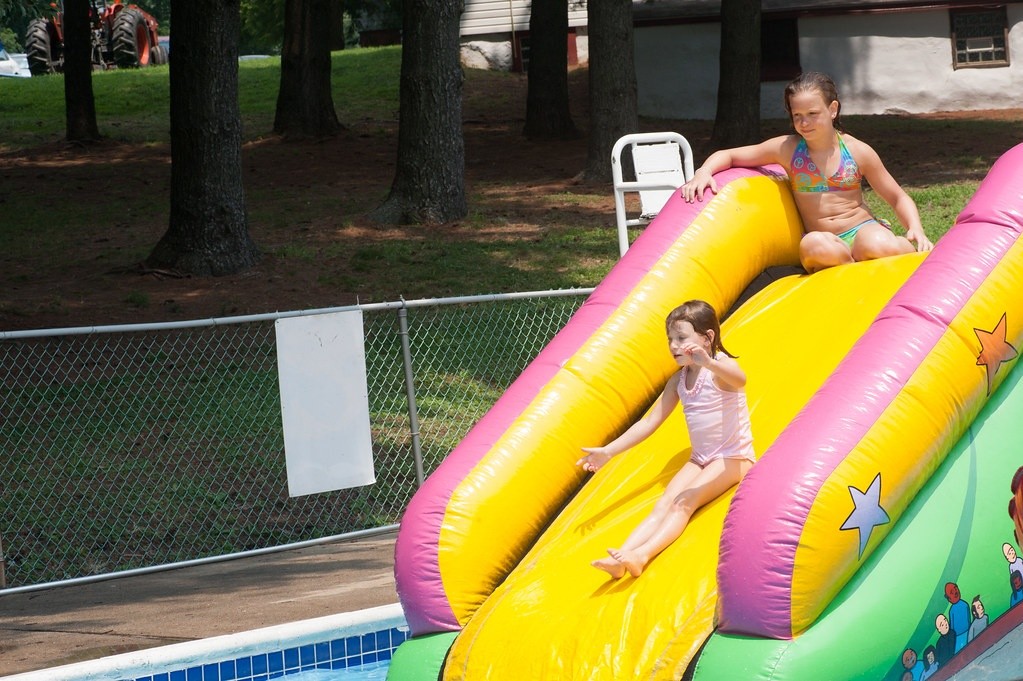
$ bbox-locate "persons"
[681,74,935,275]
[578,299,755,579]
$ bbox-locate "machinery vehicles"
[25,1,159,75]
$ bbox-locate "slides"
[443,235,934,681]
